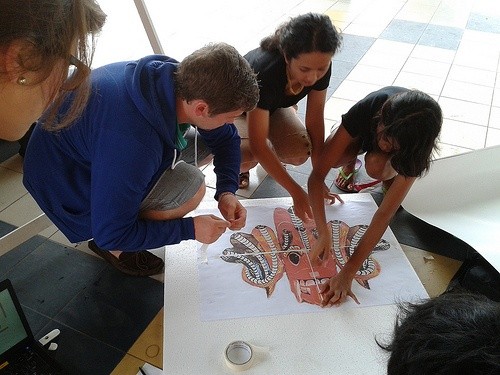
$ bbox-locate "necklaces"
[286,68,304,95]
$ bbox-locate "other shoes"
[88,239,164,276]
[238,172,249,188]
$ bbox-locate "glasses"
[61,51,90,92]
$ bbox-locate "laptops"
[0,279,63,375]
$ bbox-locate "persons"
[374,292,500,375]
[234,13,345,224]
[0,0,107,142]
[307,86,443,309]
[23,42,260,276]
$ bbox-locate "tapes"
[222,338,255,370]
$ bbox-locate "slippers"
[334,158,362,192]
